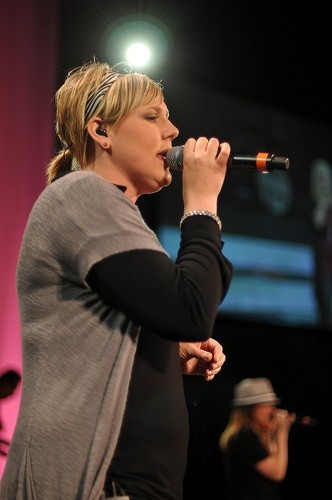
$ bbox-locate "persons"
[1,61,234,499]
[216,379,290,500]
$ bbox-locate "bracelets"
[180,210,222,230]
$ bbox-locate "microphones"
[268,410,312,425]
[166,147,291,174]
[0,370,22,400]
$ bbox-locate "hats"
[230,376,277,410]
